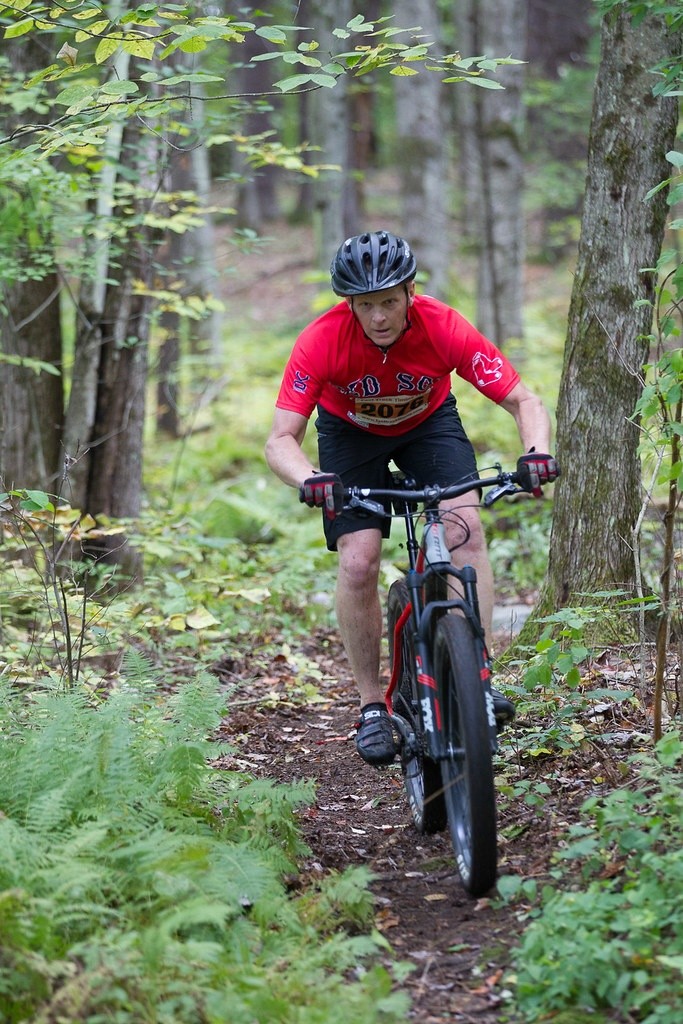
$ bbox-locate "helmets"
[330,230,417,297]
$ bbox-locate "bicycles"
[301,456,567,902]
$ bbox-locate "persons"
[263,230,558,767]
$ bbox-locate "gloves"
[516,446,559,498]
[299,470,344,520]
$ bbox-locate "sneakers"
[490,687,516,735]
[354,702,395,765]
